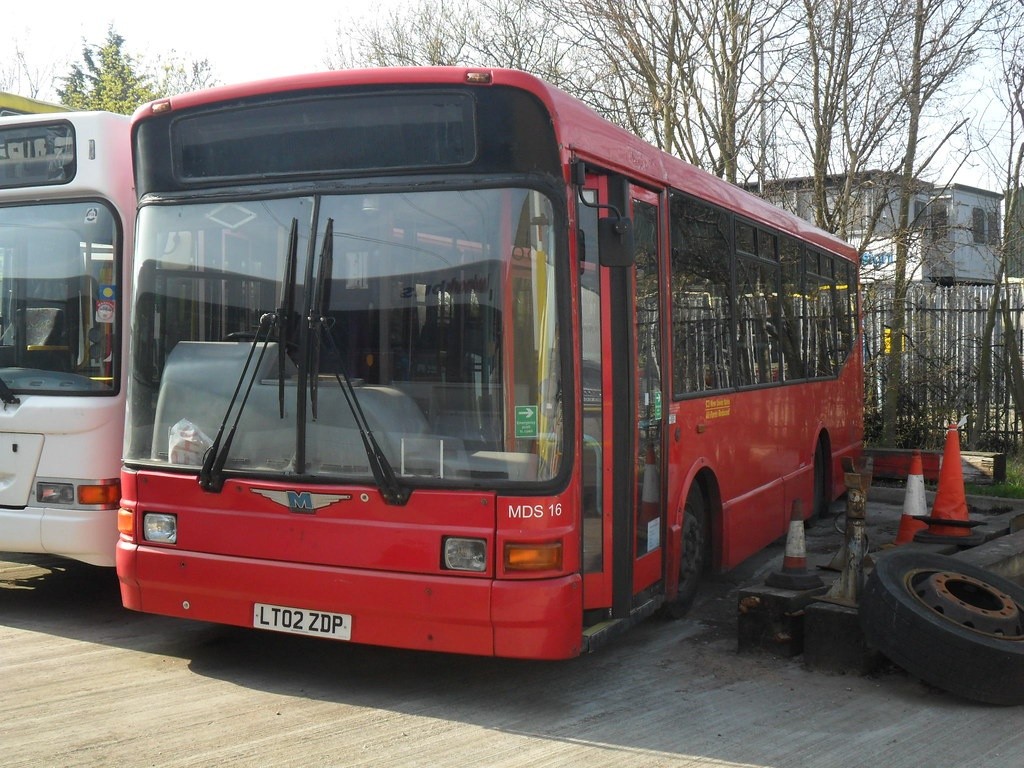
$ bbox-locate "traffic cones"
[912,424,986,545]
[764,498,820,591]
[636,445,662,546]
[879,448,930,552]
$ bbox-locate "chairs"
[0,309,68,346]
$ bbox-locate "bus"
[0,91,71,116]
[114,68,863,664]
[0,109,603,600]
